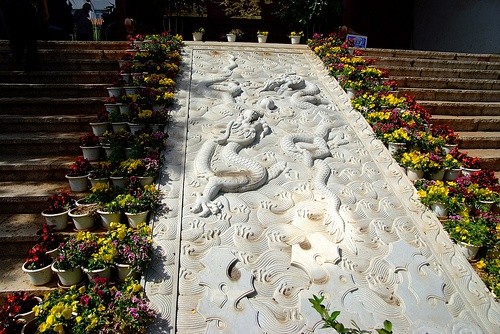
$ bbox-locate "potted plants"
[193,32,202,41]
[257,35,268,43]
[226,34,236,42]
[290,35,301,46]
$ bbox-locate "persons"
[0,0,50,73]
[74,4,95,42]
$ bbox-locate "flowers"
[307,32,500,300]
[231,29,244,37]
[257,30,269,35]
[0,30,184,334]
[290,31,306,38]
[198,27,205,33]
